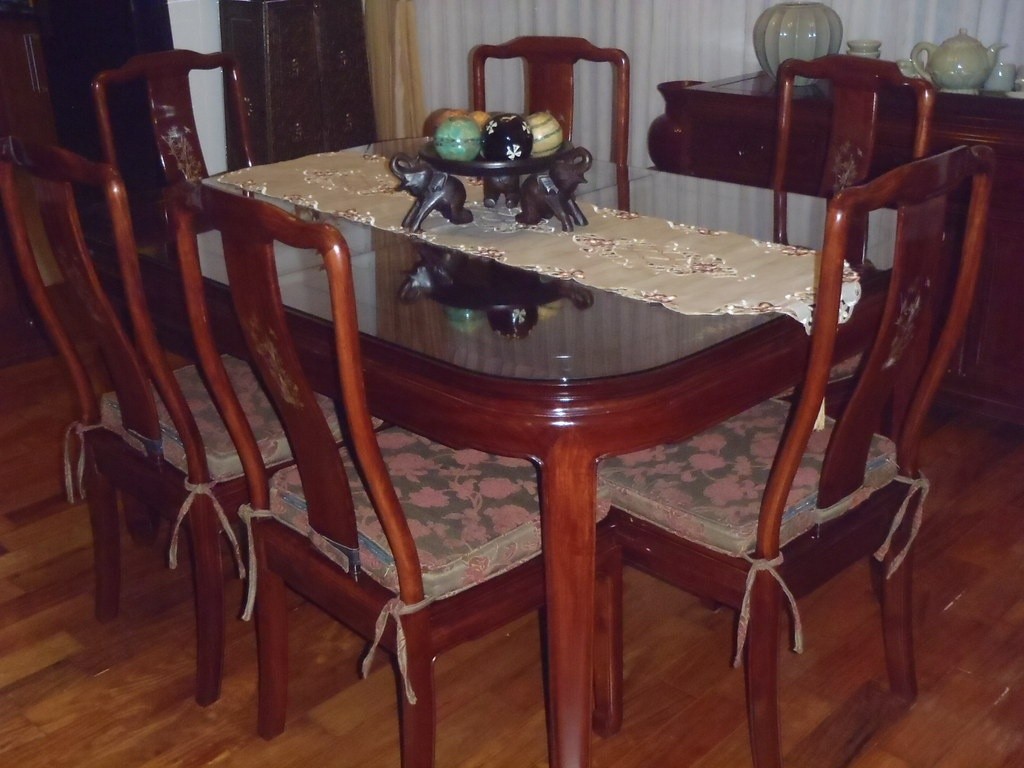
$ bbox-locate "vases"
[752,3,843,86]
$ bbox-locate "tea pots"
[911,28,1008,95]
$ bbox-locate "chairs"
[594,142,997,768]
[92,48,257,194]
[159,189,610,768]
[774,56,936,196]
[471,35,630,166]
[0,135,397,706]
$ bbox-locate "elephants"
[395,242,596,314]
[386,150,475,235]
[514,142,593,232]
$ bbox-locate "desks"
[71,140,901,768]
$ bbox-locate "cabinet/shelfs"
[649,69,1024,431]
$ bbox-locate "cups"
[845,38,881,61]
[984,61,1015,91]
[897,60,923,79]
